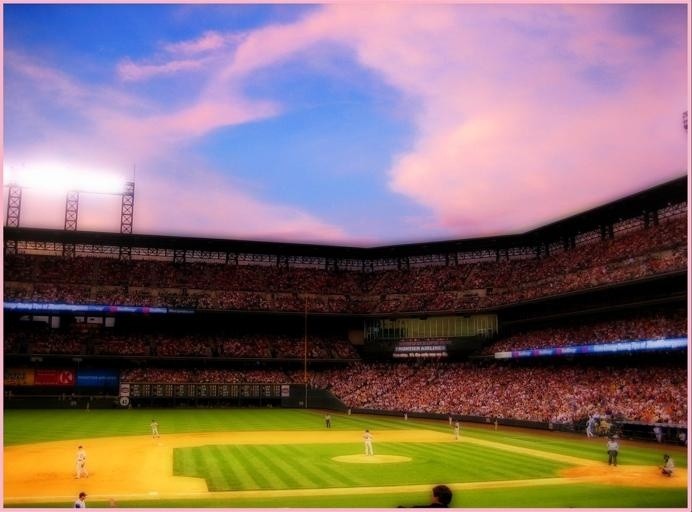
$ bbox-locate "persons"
[659,454,674,476]
[74,493,87,508]
[75,445,88,478]
[398,486,452,507]
[607,437,618,467]
[362,428,374,456]
